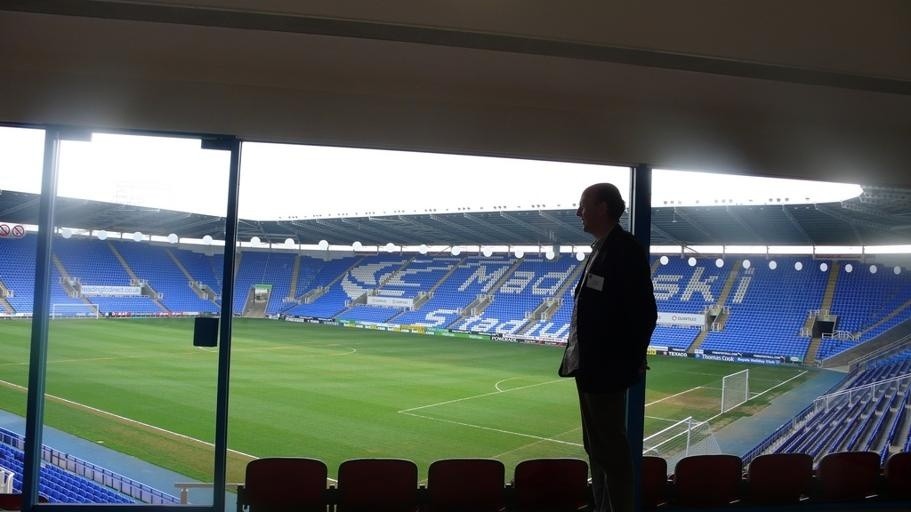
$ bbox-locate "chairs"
[0,444,139,505]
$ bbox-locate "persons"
[558,183,658,512]
